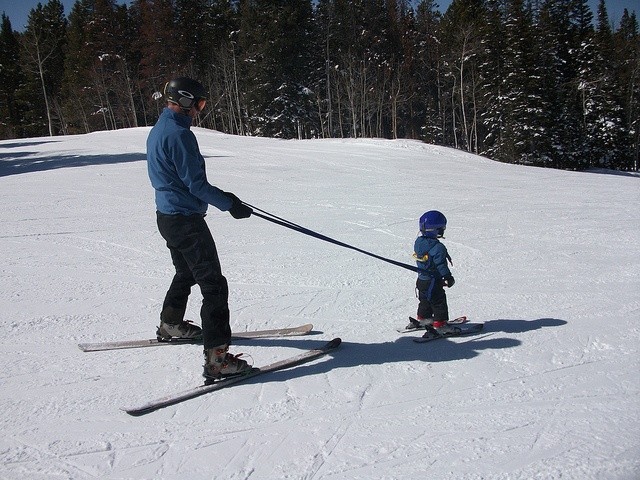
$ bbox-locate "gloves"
[441,279,448,286]
[225,192,242,204]
[229,204,253,219]
[447,276,455,288]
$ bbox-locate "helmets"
[419,210,447,239]
[164,77,209,113]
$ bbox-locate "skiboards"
[396,316,483,344]
[77,324,341,414]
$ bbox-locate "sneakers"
[433,321,455,335]
[156,320,202,340]
[417,315,434,326]
[202,345,251,375]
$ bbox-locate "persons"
[145,77,253,379]
[414,210,463,335]
